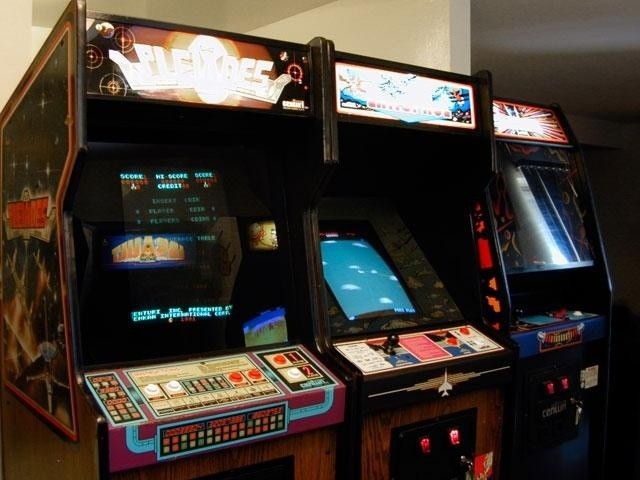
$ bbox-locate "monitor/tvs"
[489,161,595,275]
[317,218,423,321]
[119,157,237,323]
